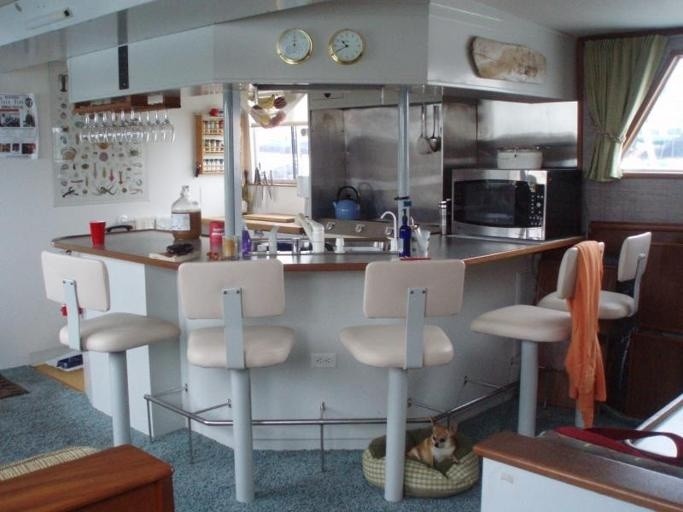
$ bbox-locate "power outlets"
[311,353,336,369]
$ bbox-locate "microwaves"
[448,167,580,241]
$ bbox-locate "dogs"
[406,416,461,468]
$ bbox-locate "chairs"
[41,250,181,448]
[339,259,466,502]
[470,242,605,436]
[538,231,652,430]
[177,259,295,502]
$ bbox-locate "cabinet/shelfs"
[195,114,225,174]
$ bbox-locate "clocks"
[276,27,313,65]
[328,28,365,65]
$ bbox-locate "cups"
[415,230,431,256]
[90,221,106,249]
[221,234,239,261]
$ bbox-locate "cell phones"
[167,243,194,253]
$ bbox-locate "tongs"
[261,172,272,199]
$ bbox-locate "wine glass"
[80,107,175,143]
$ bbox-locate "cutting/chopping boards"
[243,213,298,222]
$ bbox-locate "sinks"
[329,236,391,249]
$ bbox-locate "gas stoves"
[319,216,440,238]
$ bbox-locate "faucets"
[380,208,402,239]
[292,211,327,253]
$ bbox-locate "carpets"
[0,365,646,512]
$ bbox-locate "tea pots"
[332,186,365,221]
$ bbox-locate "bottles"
[210,221,225,254]
[242,187,248,214]
[204,120,225,171]
[440,201,448,235]
[170,185,202,239]
[241,229,251,254]
[399,214,411,257]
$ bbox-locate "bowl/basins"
[495,147,545,169]
